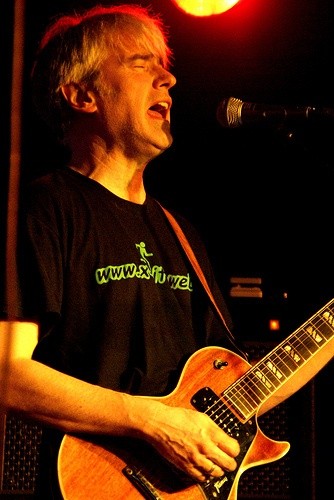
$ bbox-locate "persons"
[1,6,248,500]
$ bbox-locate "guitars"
[56,296,334,500]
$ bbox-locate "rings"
[206,464,216,474]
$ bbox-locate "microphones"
[216,96,334,128]
